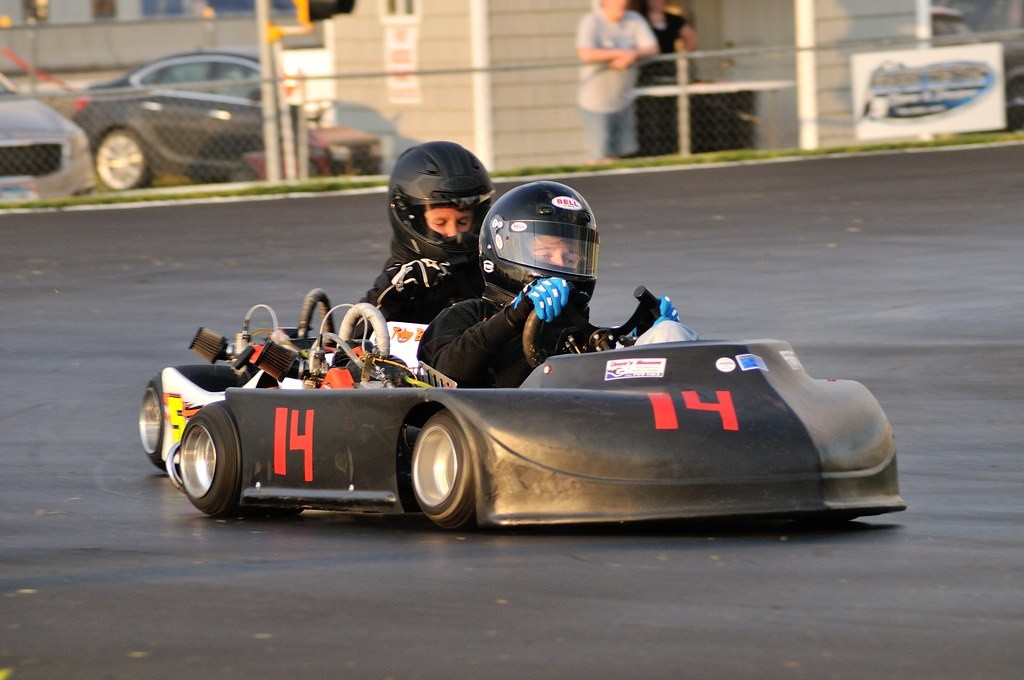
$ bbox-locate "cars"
[0,69,98,201]
[73,52,396,193]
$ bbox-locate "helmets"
[388,141,495,263]
[479,181,600,312]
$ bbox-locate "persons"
[576,0,697,166]
[353,141,495,340]
[417,181,679,388]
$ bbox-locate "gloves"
[377,258,451,315]
[632,295,680,337]
[514,277,570,322]
